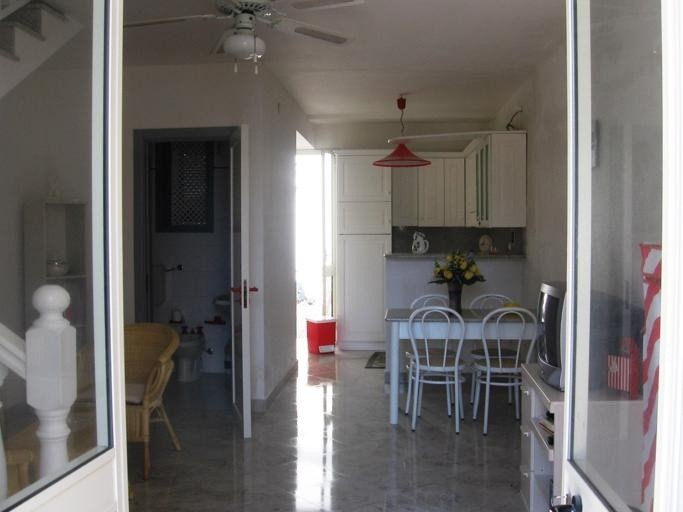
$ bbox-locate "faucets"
[189,327,197,334]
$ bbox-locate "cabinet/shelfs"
[519,362,643,512]
[381,253,523,309]
[390,129,526,227]
[22,197,90,342]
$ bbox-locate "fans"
[122,0,366,46]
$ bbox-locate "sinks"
[173,332,206,360]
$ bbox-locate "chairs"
[4,320,181,497]
[405,292,540,437]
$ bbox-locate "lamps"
[222,13,266,74]
[372,96,432,167]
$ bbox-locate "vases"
[446,281,464,316]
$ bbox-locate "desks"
[384,307,542,425]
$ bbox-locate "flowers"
[427,253,486,287]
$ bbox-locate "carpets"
[364,350,385,368]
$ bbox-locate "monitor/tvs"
[536,281,645,391]
